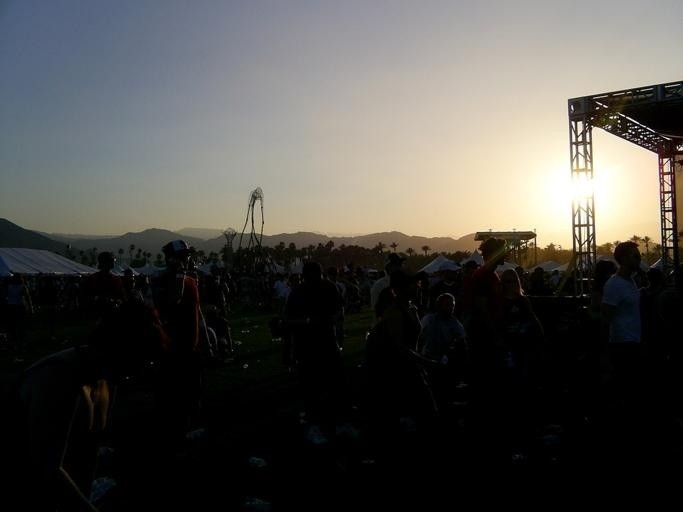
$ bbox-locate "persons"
[1,241,683,512]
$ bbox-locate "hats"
[162,240,196,253]
